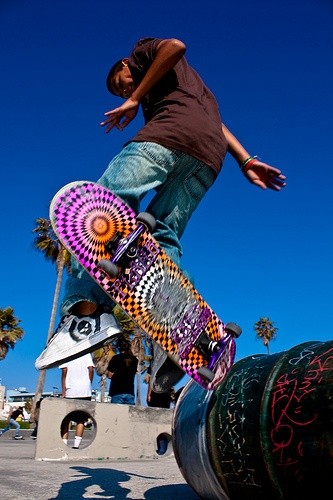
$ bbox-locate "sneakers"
[0,431,3,437]
[150,339,186,393]
[15,435,23,438]
[34,312,123,370]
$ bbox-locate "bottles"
[104,396,111,402]
[91,390,96,401]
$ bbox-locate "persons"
[34,36,286,395]
[0,406,24,440]
[30,397,44,439]
[105,335,139,404]
[58,352,96,449]
[142,341,174,455]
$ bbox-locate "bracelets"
[241,154,261,173]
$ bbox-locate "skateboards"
[50,180,244,390]
